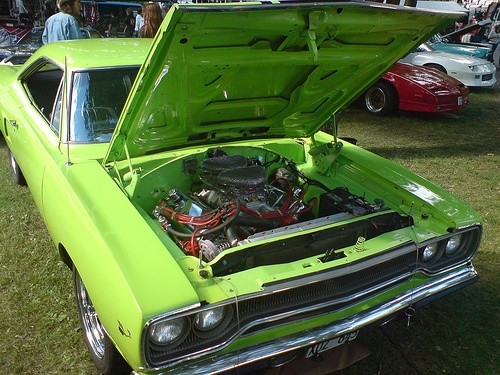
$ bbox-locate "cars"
[0,26,105,64]
[0,1,484,374]
[398,19,498,90]
[362,61,470,118]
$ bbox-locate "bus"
[81,1,143,37]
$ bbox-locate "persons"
[135,3,164,38]
[107,11,119,38]
[487,24,500,65]
[42,0,81,44]
[134,7,145,37]
[124,8,136,38]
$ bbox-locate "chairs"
[44,78,123,121]
